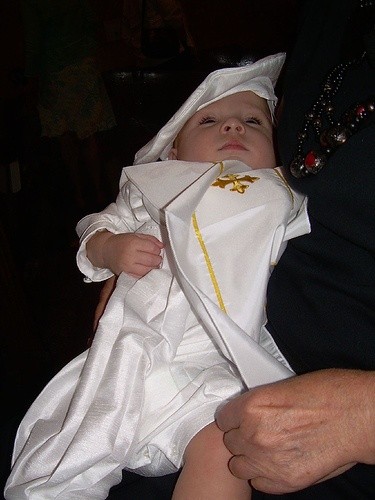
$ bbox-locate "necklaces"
[288,47,375,179]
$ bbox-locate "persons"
[18,0,116,208]
[3,52,310,500]
[119,0,197,68]
[0,0,375,500]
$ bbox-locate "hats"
[134,53,286,165]
